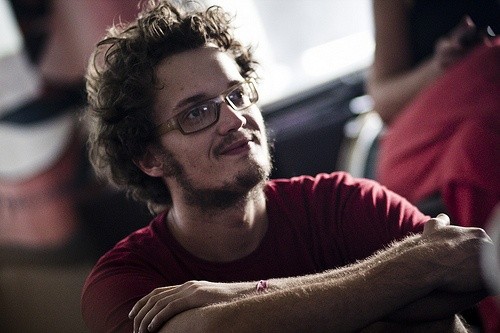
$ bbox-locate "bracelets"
[256,280,268,293]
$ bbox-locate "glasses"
[146,80,259,142]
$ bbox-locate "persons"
[368,0,500,127]
[82,3,500,333]
